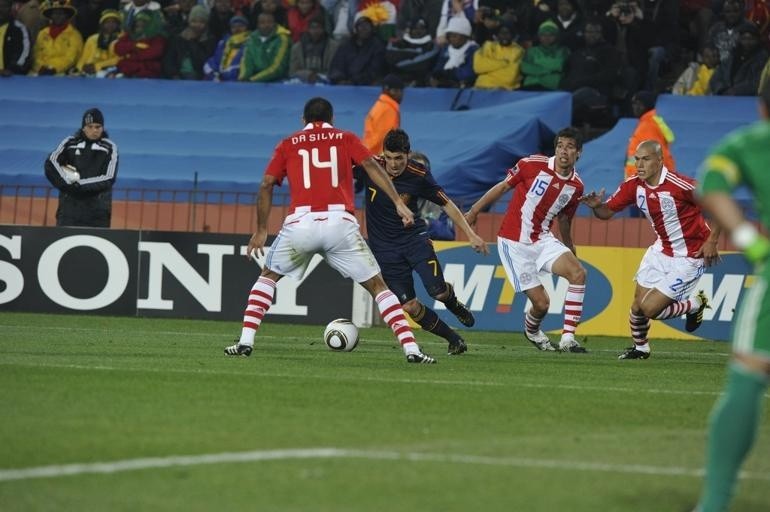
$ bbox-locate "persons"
[463,127,587,354]
[224,97,437,363]
[576,143,721,360]
[1,1,769,128]
[42,108,119,227]
[694,63,770,511]
[353,73,403,238]
[352,128,489,355]
[625,90,677,219]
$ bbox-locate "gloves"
[735,223,768,287]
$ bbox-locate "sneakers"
[451,304,475,327]
[561,340,587,354]
[524,327,557,353]
[406,348,437,363]
[224,343,253,359]
[618,345,652,361]
[448,339,468,359]
[685,292,708,331]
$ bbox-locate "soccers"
[323,318,358,351]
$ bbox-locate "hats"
[43,0,603,36]
[737,20,763,39]
[82,107,105,126]
